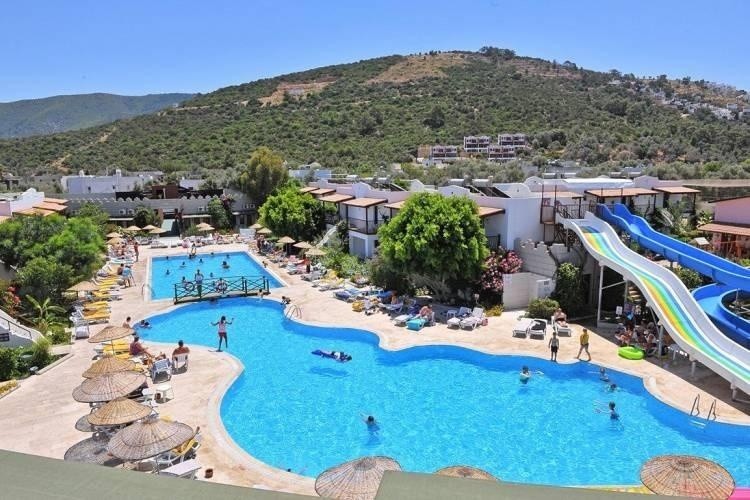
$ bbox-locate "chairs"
[616,322,689,361]
[76,254,134,324]
[149,353,188,384]
[513,313,571,339]
[260,245,484,331]
[134,233,242,248]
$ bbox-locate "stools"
[155,385,174,402]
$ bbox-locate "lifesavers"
[184,282,195,292]
[217,283,228,292]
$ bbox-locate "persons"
[520,364,529,377]
[143,321,150,327]
[362,415,376,423]
[573,328,592,361]
[599,367,609,382]
[611,383,617,389]
[210,315,234,351]
[313,348,352,362]
[129,336,154,360]
[553,307,568,329]
[411,303,433,322]
[205,467,215,479]
[547,331,559,363]
[147,352,167,365]
[123,317,132,329]
[391,293,400,306]
[172,426,201,454]
[139,319,145,327]
[305,255,312,274]
[194,268,205,297]
[171,340,190,363]
[594,400,617,420]
[612,308,657,355]
[115,234,284,290]
[258,288,264,299]
[280,295,291,306]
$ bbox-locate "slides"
[555,203,750,396]
